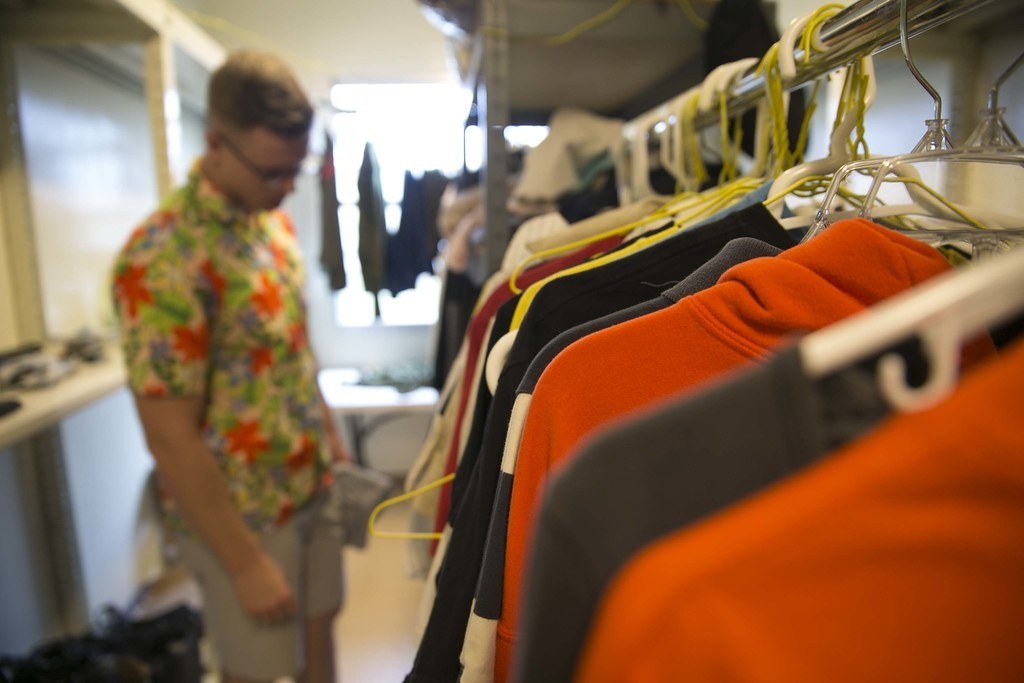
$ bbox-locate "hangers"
[369,473,456,540]
[508,0,1024,413]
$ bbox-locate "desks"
[316,369,440,470]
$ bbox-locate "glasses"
[220,134,300,181]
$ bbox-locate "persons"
[109,47,354,681]
